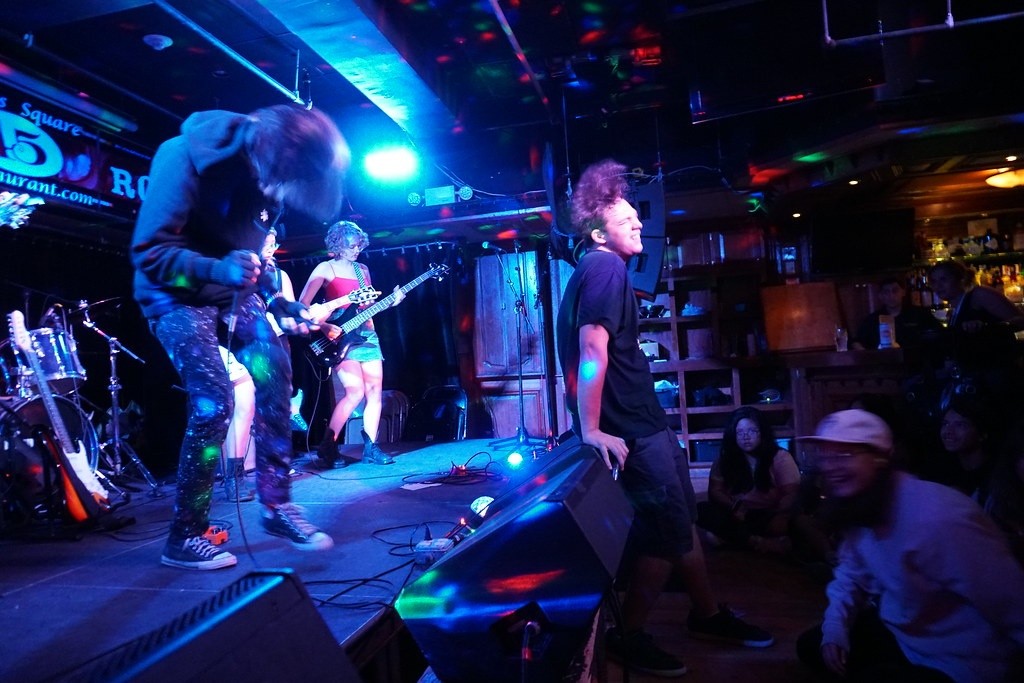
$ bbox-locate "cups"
[834,328,847,351]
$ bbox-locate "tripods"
[82,311,165,499]
[486,251,551,460]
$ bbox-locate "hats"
[792,409,898,455]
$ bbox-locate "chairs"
[343,384,469,446]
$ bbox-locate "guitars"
[7,310,114,523]
[301,262,455,367]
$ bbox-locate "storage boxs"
[773,438,792,452]
[691,441,721,462]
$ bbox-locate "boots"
[361,429,394,465]
[317,428,347,468]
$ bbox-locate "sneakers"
[161,536,237,570]
[686,603,774,647]
[260,502,334,553]
[604,626,687,677]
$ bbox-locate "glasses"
[803,447,872,467]
[735,429,760,440]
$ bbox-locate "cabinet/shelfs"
[636,184,804,472]
[478,381,557,437]
[473,251,547,378]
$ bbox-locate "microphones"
[482,241,501,250]
[228,250,255,341]
[39,304,56,326]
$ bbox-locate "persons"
[692,406,840,585]
[795,409,1024,683]
[130,104,406,572]
[933,392,1024,567]
[557,158,775,677]
[847,260,1024,350]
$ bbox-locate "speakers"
[58,568,372,683]
[621,182,667,302]
[393,435,636,683]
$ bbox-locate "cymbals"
[64,297,119,326]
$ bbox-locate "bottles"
[914,229,1024,325]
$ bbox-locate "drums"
[0,394,99,521]
[0,327,87,399]
